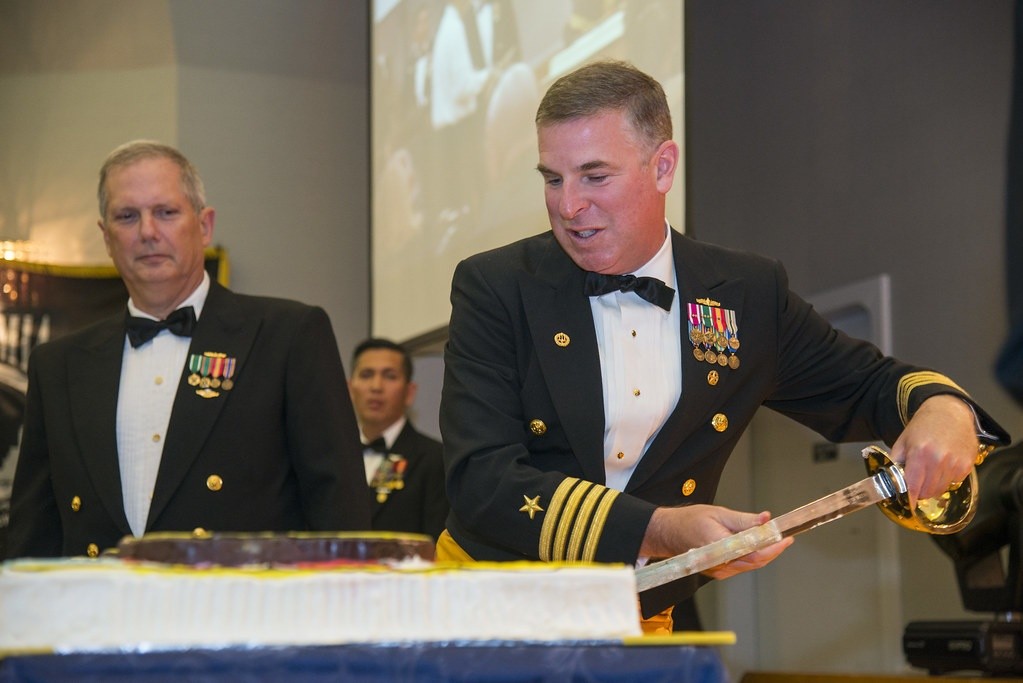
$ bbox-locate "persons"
[432,57,1013,634]
[7,140,372,558]
[353,339,447,545]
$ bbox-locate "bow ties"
[125,306,197,349]
[361,437,385,453]
[583,271,676,312]
